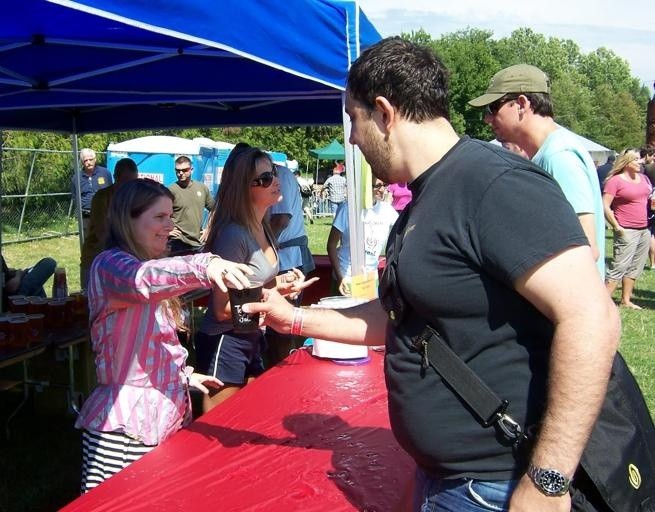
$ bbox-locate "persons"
[72,147,112,249]
[385,181,412,215]
[0,252,57,298]
[468,63,655,311]
[71,178,256,497]
[167,156,216,257]
[194,143,319,415]
[320,167,347,218]
[269,165,315,361]
[79,158,139,289]
[328,174,402,298]
[242,37,622,512]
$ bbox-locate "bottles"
[51,267,67,298]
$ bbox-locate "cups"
[228,280,267,336]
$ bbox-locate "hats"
[467,63,551,108]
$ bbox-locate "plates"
[0,313,45,324]
[7,293,74,306]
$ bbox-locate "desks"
[299,253,387,306]
[59,342,430,512]
[0,288,212,441]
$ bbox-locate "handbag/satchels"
[571,349,655,512]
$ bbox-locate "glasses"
[251,163,279,188]
[175,167,190,172]
[487,97,518,113]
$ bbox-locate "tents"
[306,139,346,183]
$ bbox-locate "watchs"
[526,460,572,497]
